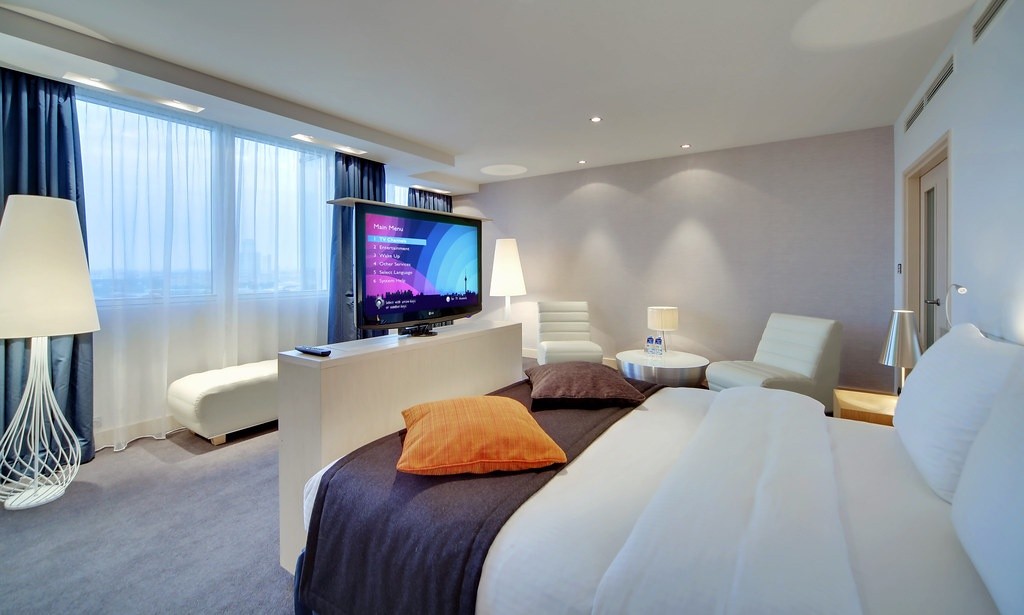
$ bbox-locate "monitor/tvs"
[352,202,482,337]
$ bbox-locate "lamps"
[946,283,967,329]
[879,310,922,391]
[0,195,101,511]
[647,306,679,352]
[489,239,527,323]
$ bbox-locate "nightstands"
[832,385,899,427]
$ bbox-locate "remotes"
[296,345,331,357]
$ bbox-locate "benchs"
[168,358,279,446]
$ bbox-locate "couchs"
[535,301,603,366]
[705,313,843,413]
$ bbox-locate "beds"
[300,377,1002,615]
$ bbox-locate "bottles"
[646,336,654,359]
[655,335,662,360]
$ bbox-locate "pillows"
[893,323,1024,504]
[950,345,1024,615]
[396,396,568,477]
[524,361,647,402]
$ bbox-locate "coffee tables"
[615,349,710,387]
